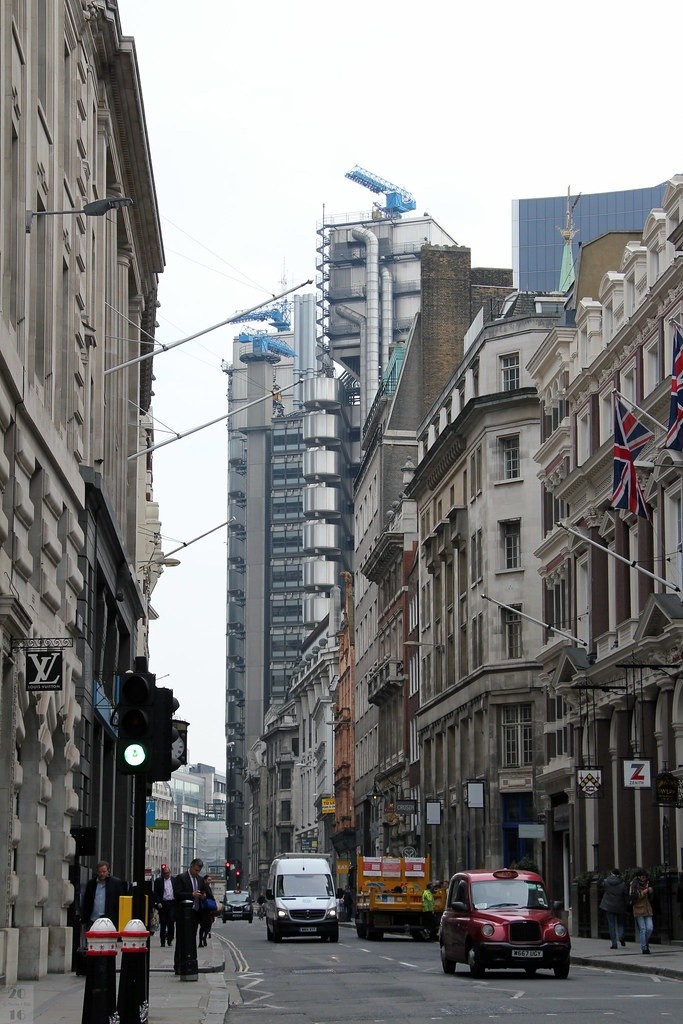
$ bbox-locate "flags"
[665,326,683,450]
[610,396,656,520]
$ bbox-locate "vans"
[220,891,256,924]
[264,859,345,942]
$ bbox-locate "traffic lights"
[226,863,230,872]
[236,871,240,883]
[161,864,166,872]
[116,672,154,776]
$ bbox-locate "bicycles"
[257,906,265,920]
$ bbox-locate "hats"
[611,869,619,875]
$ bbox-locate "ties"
[193,878,199,910]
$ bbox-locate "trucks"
[354,851,446,943]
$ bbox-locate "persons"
[154,867,176,946]
[435,879,449,890]
[257,894,266,919]
[199,875,217,947]
[80,861,122,935]
[600,869,629,949]
[337,885,352,922]
[174,859,207,974]
[422,882,437,941]
[629,869,654,954]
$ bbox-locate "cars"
[439,869,571,980]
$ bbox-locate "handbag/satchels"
[201,898,217,911]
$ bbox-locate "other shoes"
[610,945,617,949]
[198,943,204,948]
[209,933,211,938]
[619,937,625,946]
[204,937,207,946]
[642,949,650,954]
[160,942,165,947]
[167,941,172,946]
